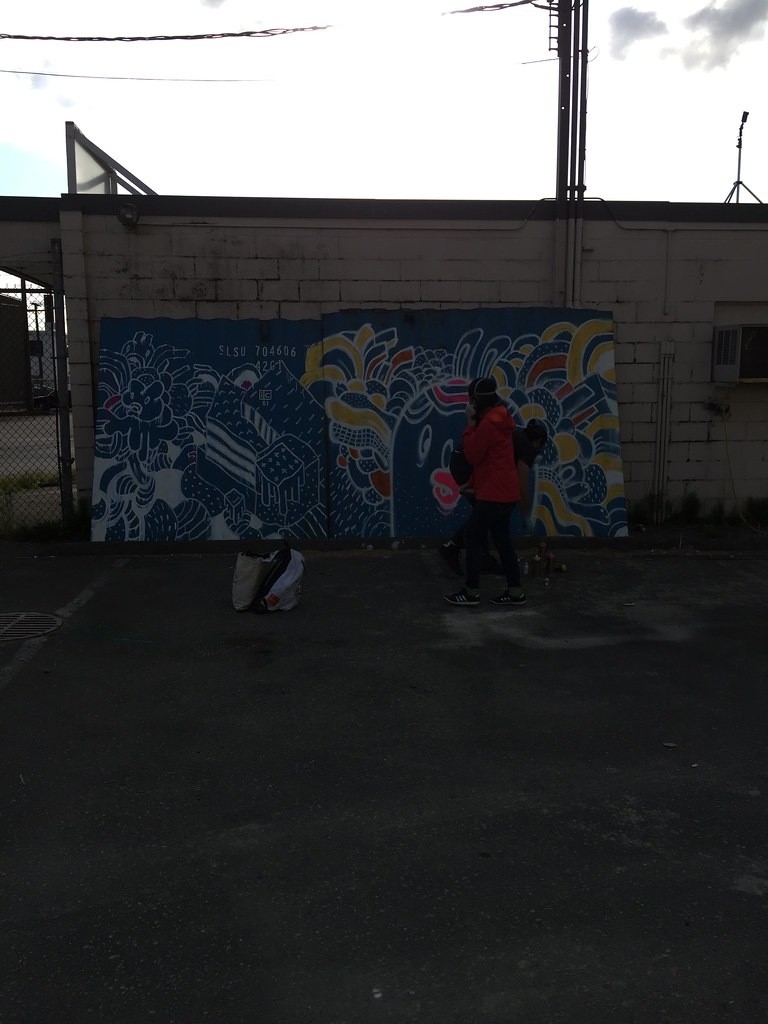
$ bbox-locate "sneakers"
[442,591,481,605]
[489,593,528,605]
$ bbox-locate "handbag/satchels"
[232,549,264,611]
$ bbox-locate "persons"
[439,418,549,578]
[440,377,528,606]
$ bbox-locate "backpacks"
[259,547,305,613]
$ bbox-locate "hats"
[468,376,498,401]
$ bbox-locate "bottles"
[523,541,568,576]
